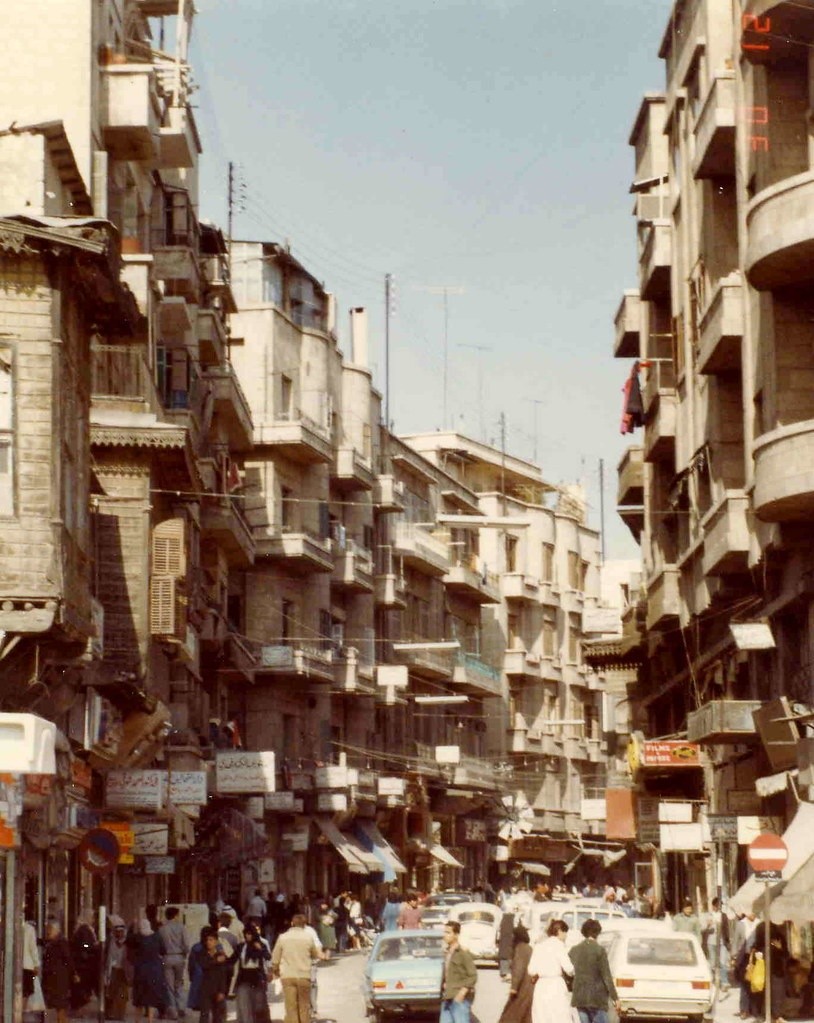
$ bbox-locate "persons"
[0,870,814,1023]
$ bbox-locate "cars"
[421,892,478,933]
[364,929,451,1023]
[526,893,629,954]
[600,931,716,1022]
[447,901,509,964]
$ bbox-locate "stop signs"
[746,833,790,876]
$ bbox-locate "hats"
[138,919,154,937]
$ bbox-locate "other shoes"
[741,1011,749,1019]
[735,1011,744,1016]
[776,1017,787,1023]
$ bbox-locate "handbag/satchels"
[26,976,45,1013]
[744,954,755,981]
[751,959,765,993]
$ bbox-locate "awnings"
[516,860,551,877]
[313,817,465,886]
